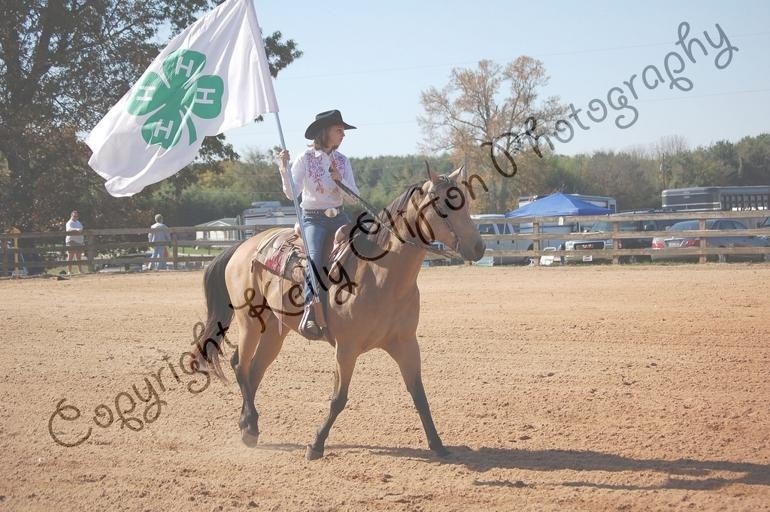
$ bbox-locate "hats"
[305,110,357,139]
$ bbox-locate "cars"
[98,251,151,273]
[430,187,770,265]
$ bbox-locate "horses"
[186,159,487,461]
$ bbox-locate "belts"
[303,206,344,218]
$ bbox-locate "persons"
[280,109,359,332]
[65,209,85,275]
[148,213,171,270]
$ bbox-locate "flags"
[84,0,280,196]
